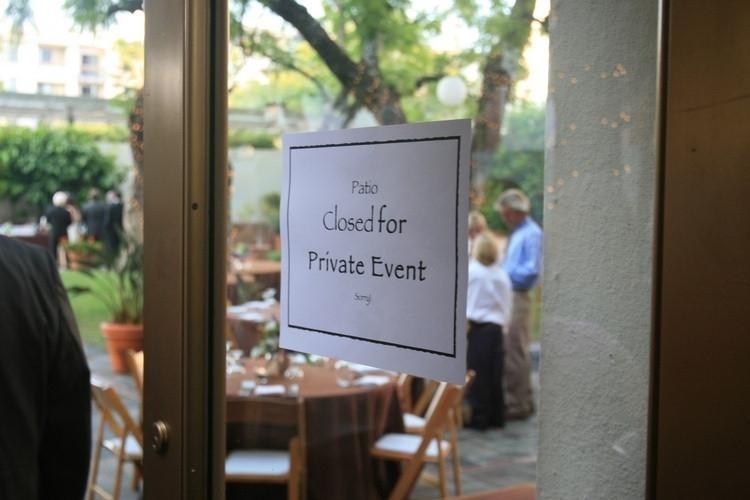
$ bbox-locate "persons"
[38,186,124,271]
[500,188,542,420]
[468,211,488,241]
[466,234,512,431]
[1,230,93,500]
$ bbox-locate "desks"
[226,245,400,500]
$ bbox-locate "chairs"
[370,369,478,499]
[122,348,145,394]
[85,384,143,499]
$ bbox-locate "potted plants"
[57,230,144,373]
